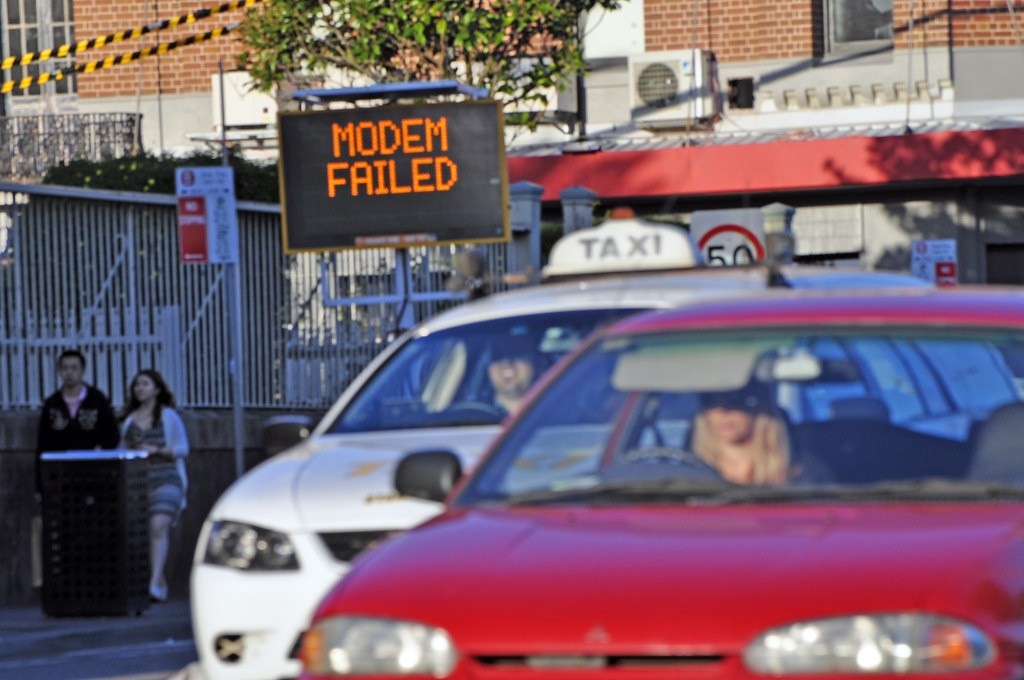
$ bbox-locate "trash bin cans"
[41,451,151,618]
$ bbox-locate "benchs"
[795,419,968,485]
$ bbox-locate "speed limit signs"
[696,223,767,270]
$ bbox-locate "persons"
[34,350,120,488]
[121,370,191,602]
[692,376,794,488]
[487,337,542,415]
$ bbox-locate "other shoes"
[150,586,169,603]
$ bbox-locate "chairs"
[963,401,1024,483]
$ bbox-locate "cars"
[271,266,1024,680]
[186,205,1024,680]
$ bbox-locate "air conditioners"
[627,48,719,125]
